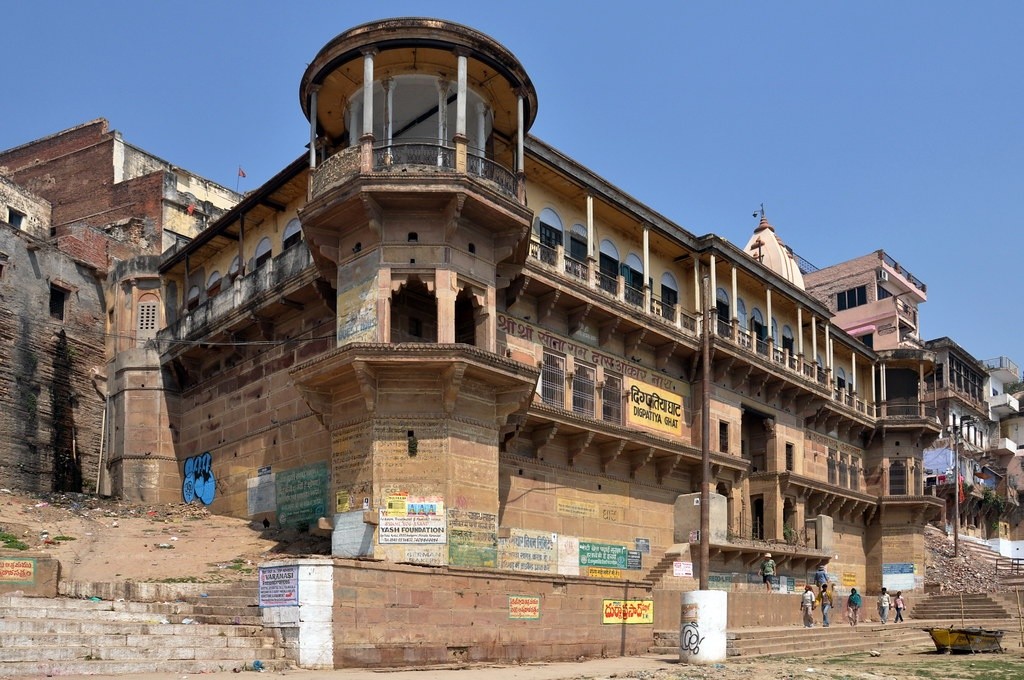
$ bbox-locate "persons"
[847,588,862,626]
[758,553,776,592]
[877,587,891,624]
[894,591,906,623]
[814,565,830,592]
[800,585,815,627]
[815,584,834,627]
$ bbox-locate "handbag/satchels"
[812,599,820,610]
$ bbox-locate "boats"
[924,623,1005,654]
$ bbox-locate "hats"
[805,585,811,590]
[765,553,771,557]
[818,566,824,570]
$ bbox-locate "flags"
[239,168,246,177]
[188,204,194,215]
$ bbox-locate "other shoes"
[900,621,903,623]
[805,624,813,628]
[823,623,829,627]
[894,621,897,623]
